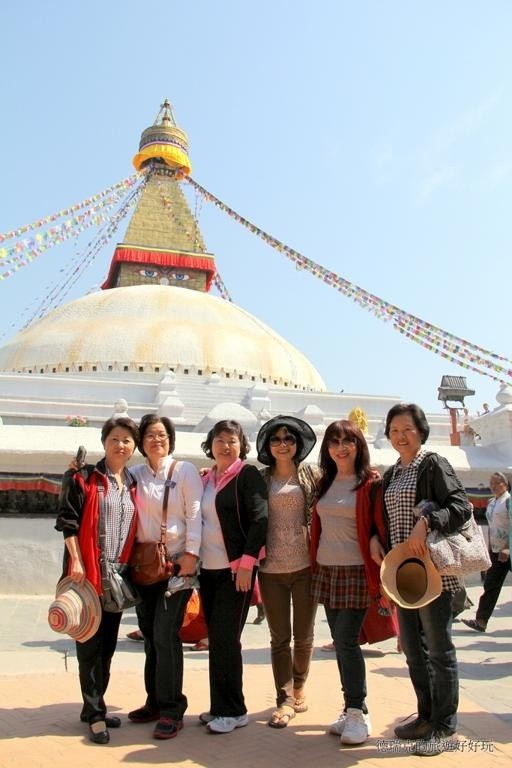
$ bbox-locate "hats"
[379,541,443,609]
[48,574,101,643]
[254,415,317,466]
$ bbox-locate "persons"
[126,628,210,652]
[70,412,204,738]
[461,471,512,632]
[53,417,139,745]
[248,571,266,625]
[369,402,468,757]
[200,414,379,728]
[320,603,404,653]
[308,420,400,745]
[197,420,269,734]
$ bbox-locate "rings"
[244,584,248,588]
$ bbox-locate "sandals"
[127,629,144,642]
[459,618,488,632]
[190,640,210,651]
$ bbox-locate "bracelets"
[185,552,199,559]
[421,514,430,534]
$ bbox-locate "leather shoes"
[87,715,109,744]
[81,708,121,727]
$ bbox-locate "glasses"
[268,436,298,448]
[327,437,358,448]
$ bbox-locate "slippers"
[269,705,296,729]
[293,695,308,714]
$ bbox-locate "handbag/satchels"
[426,516,492,576]
[130,540,174,584]
[100,558,140,612]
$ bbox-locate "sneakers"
[199,707,215,721]
[154,713,185,739]
[127,702,161,723]
[340,708,371,745]
[207,710,248,734]
[250,611,266,626]
[412,727,459,756]
[328,713,346,734]
[323,641,337,651]
[394,714,428,737]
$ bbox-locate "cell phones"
[75,446,87,465]
